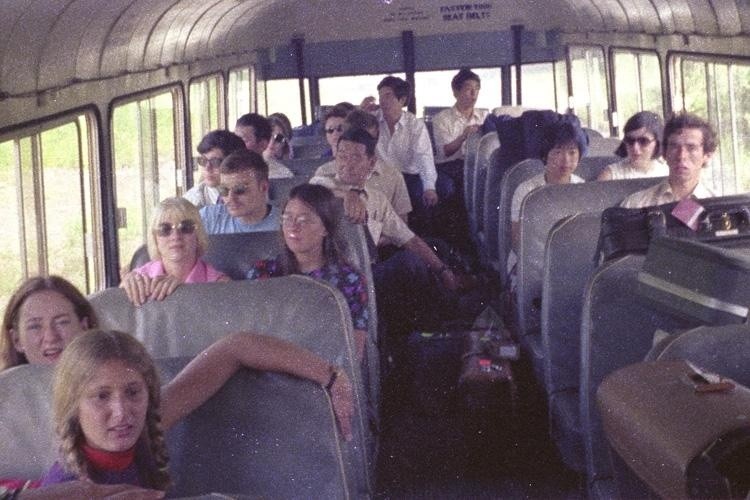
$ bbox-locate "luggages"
[594,194,750,500]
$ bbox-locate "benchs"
[0,102,400,500]
[421,102,750,500]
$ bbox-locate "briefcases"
[459,307,516,421]
[594,194,750,499]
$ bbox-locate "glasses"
[154,218,196,236]
[324,126,343,134]
[197,155,223,168]
[218,183,257,196]
[271,134,286,143]
[624,136,658,147]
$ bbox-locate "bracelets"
[326,371,338,391]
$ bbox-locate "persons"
[0,276,98,372]
[506,122,586,308]
[619,111,721,209]
[596,111,670,181]
[16,330,356,500]
